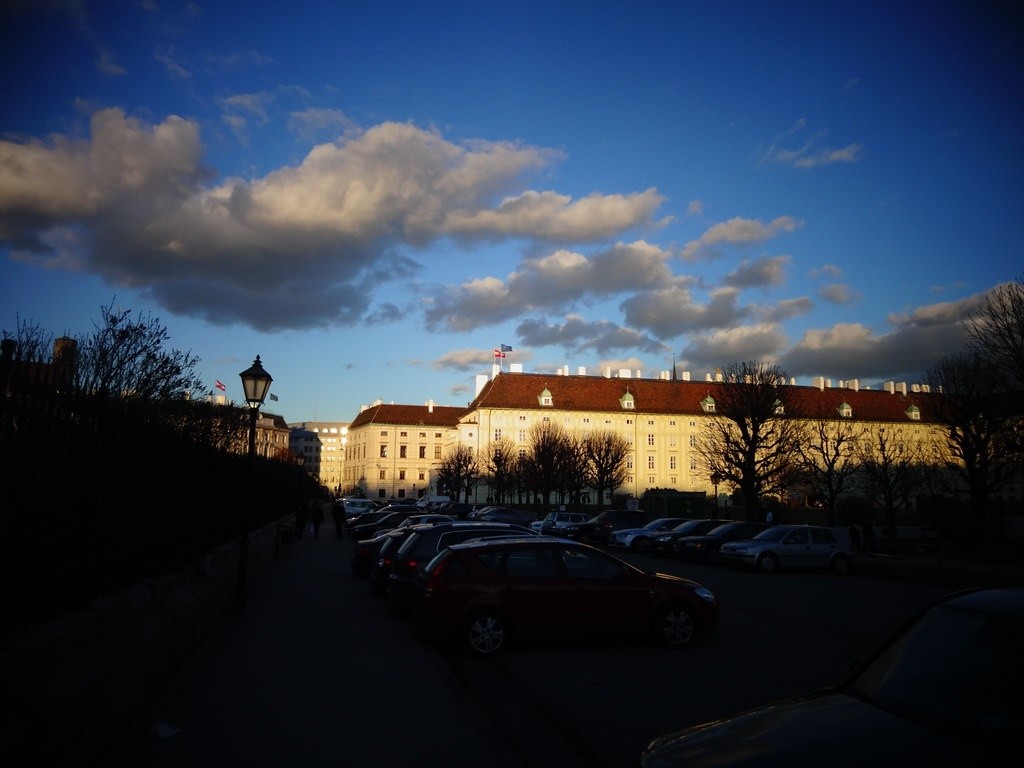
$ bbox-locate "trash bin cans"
[277,524,294,546]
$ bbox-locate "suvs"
[562,510,655,544]
[417,536,720,654]
[353,512,454,534]
[344,506,421,535]
[609,518,693,552]
[392,524,542,609]
[648,519,734,552]
[426,501,473,514]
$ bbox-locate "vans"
[542,512,594,535]
[342,499,378,513]
[415,496,450,510]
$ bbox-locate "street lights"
[238,355,272,611]
[711,470,723,518]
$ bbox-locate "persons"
[310,507,324,540]
[293,503,306,540]
[331,503,346,539]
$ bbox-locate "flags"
[501,344,513,352]
[494,349,505,358]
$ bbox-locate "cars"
[476,506,523,521]
[673,523,773,565]
[642,587,1023,768]
[720,525,842,572]
[400,498,417,505]
[529,521,544,532]
[384,499,401,506]
